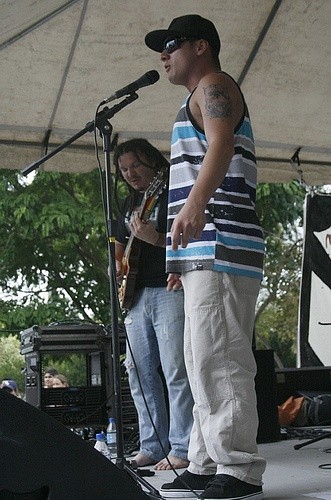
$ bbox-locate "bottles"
[106,417,117,464]
[93,434,111,460]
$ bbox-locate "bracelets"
[154,233,165,248]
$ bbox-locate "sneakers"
[196,473,264,500]
[159,470,216,497]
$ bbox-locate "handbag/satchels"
[278,396,304,424]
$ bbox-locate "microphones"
[100,70,160,105]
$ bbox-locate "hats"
[0,380,17,391]
[144,14,221,53]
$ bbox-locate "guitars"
[118,170,166,310]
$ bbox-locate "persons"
[106,139,194,469]
[43,369,69,388]
[1,381,21,398]
[144,15,265,500]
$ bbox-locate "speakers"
[0,388,151,500]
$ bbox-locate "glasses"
[160,36,206,54]
